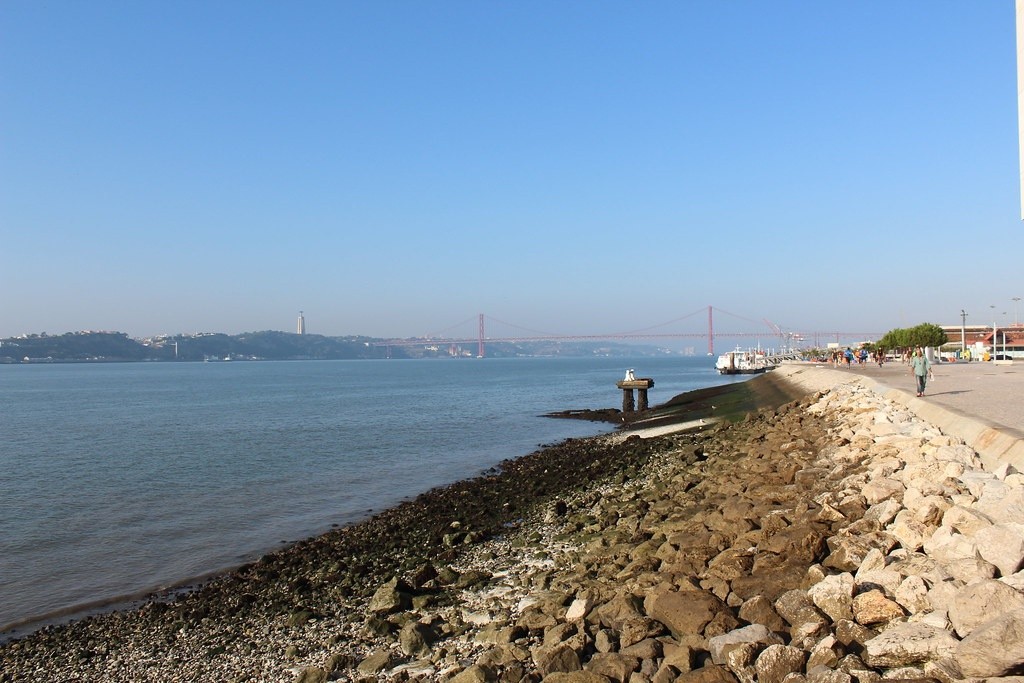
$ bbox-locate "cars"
[987,354,1013,361]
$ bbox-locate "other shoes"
[921,392,924,396]
[917,392,921,397]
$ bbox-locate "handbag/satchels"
[930,372,934,382]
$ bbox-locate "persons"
[905,344,932,397]
[832,345,885,370]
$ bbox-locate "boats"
[713,341,806,374]
